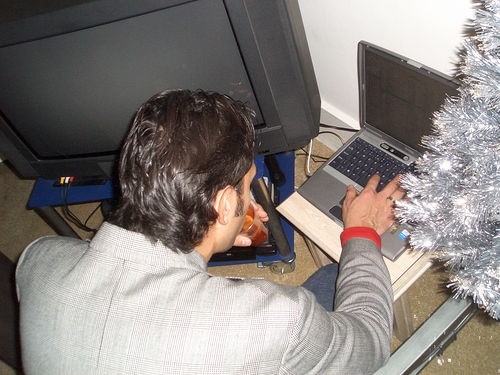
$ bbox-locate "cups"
[240,203,268,248]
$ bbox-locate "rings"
[387,197,396,206]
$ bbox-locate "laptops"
[295,40,463,261]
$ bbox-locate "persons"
[16,88,407,375]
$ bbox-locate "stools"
[301,232,414,343]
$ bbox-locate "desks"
[26,149,296,264]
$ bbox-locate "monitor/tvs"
[0,0,322,187]
